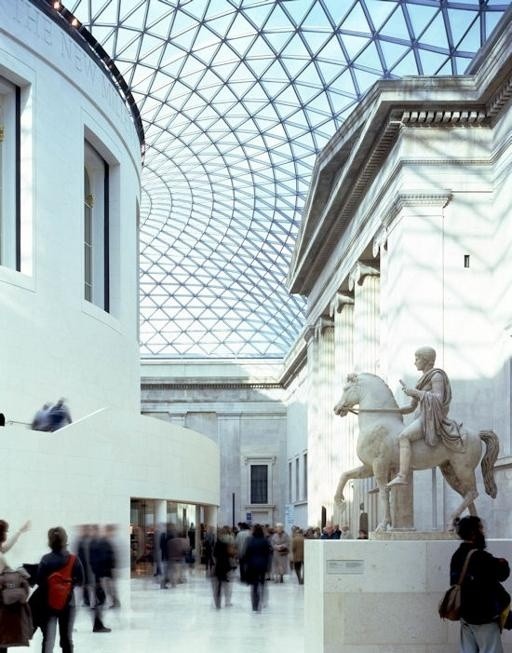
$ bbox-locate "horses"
[333,372,499,532]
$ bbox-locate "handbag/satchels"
[47,555,76,610]
[439,584,462,621]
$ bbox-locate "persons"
[0,414,6,427]
[130,520,368,612]
[31,398,74,430]
[449,516,510,653]
[0,519,121,653]
[368,346,452,494]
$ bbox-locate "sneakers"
[93,628,110,632]
